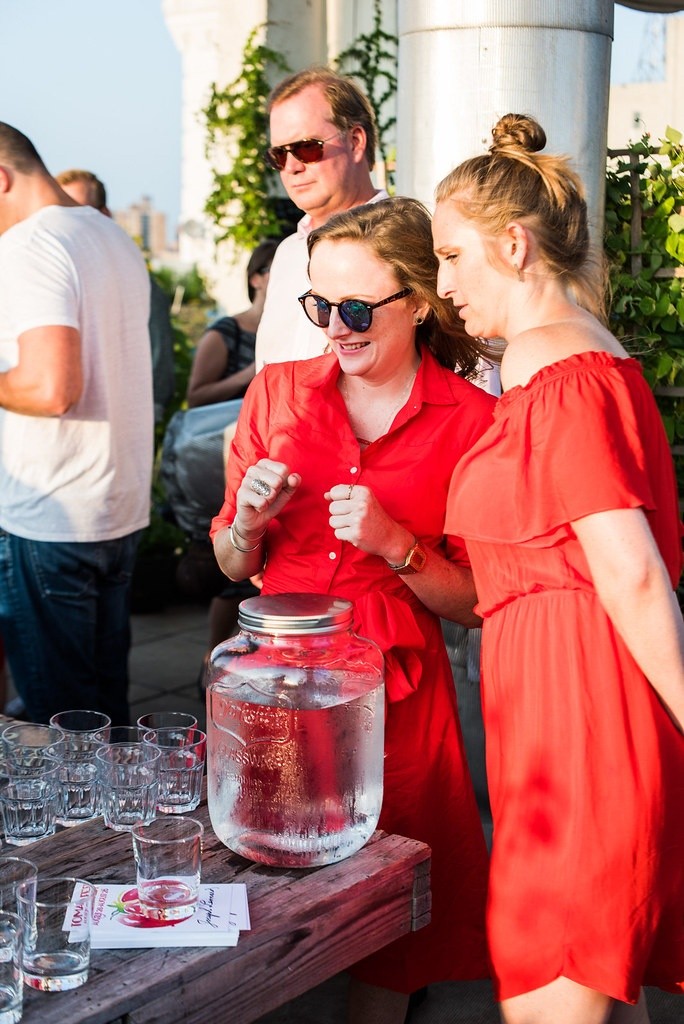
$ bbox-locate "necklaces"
[344,362,420,445]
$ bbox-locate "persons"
[0,123,156,743]
[210,193,497,1024]
[187,244,280,703]
[255,65,501,403]
[429,112,684,1022]
[5,171,173,718]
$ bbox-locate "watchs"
[386,533,426,576]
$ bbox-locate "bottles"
[204,592,386,870]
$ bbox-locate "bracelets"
[233,513,266,541]
[230,525,260,552]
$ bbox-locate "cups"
[130,815,204,921]
[0,909,26,1024]
[15,876,97,992]
[0,856,38,954]
[0,709,207,847]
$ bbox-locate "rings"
[347,484,354,500]
[250,479,271,496]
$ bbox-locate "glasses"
[297,287,414,333]
[266,133,336,172]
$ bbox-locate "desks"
[0,714,433,1024]
